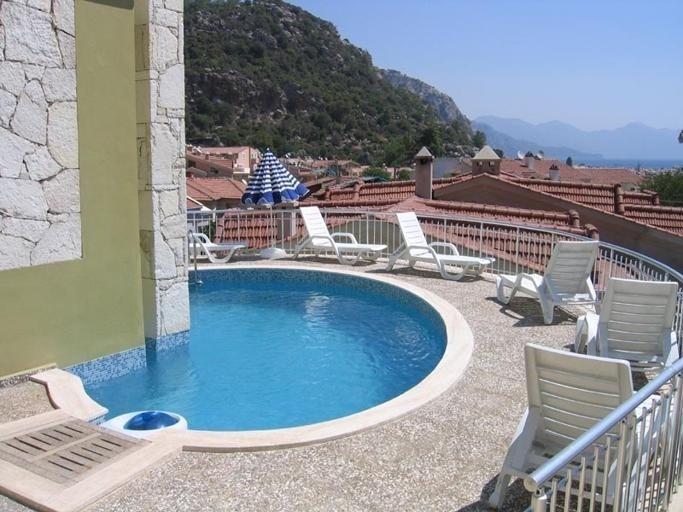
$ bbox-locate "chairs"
[292,205,387,264]
[573,276,679,389]
[487,341,679,511]
[386,211,496,281]
[494,240,597,324]
[189,232,245,264]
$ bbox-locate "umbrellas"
[240,147,310,241]
